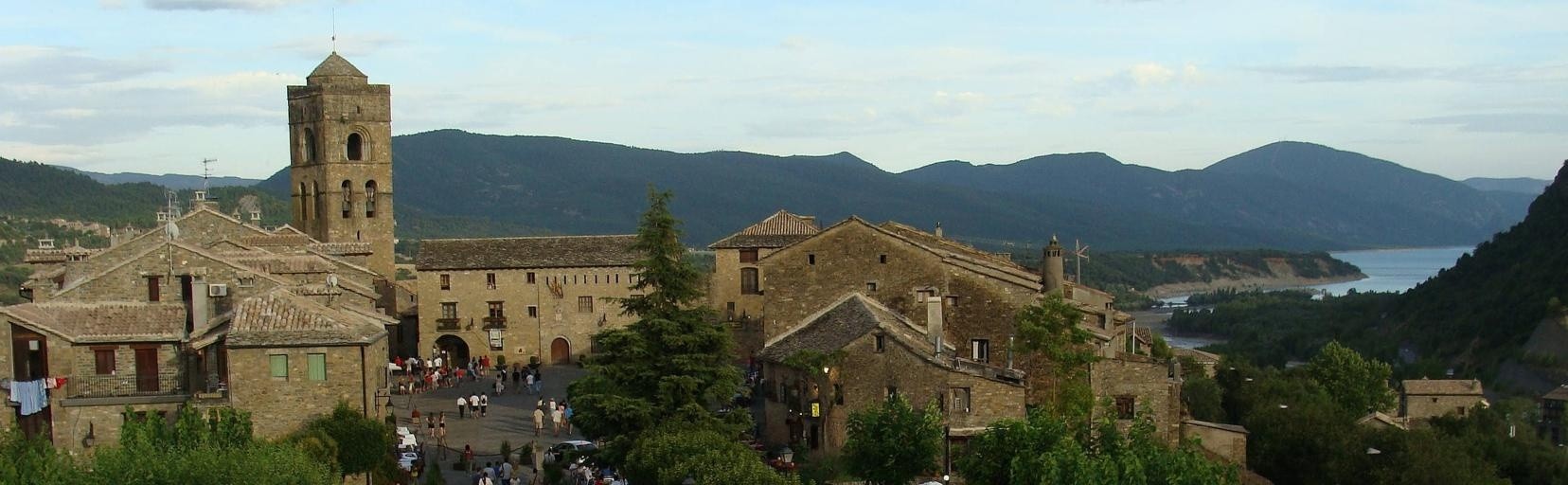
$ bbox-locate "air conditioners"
[209,284,227,296]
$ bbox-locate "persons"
[388,348,628,484]
[719,365,766,441]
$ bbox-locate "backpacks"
[481,396,486,404]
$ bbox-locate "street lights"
[775,444,796,479]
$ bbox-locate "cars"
[544,439,599,464]
